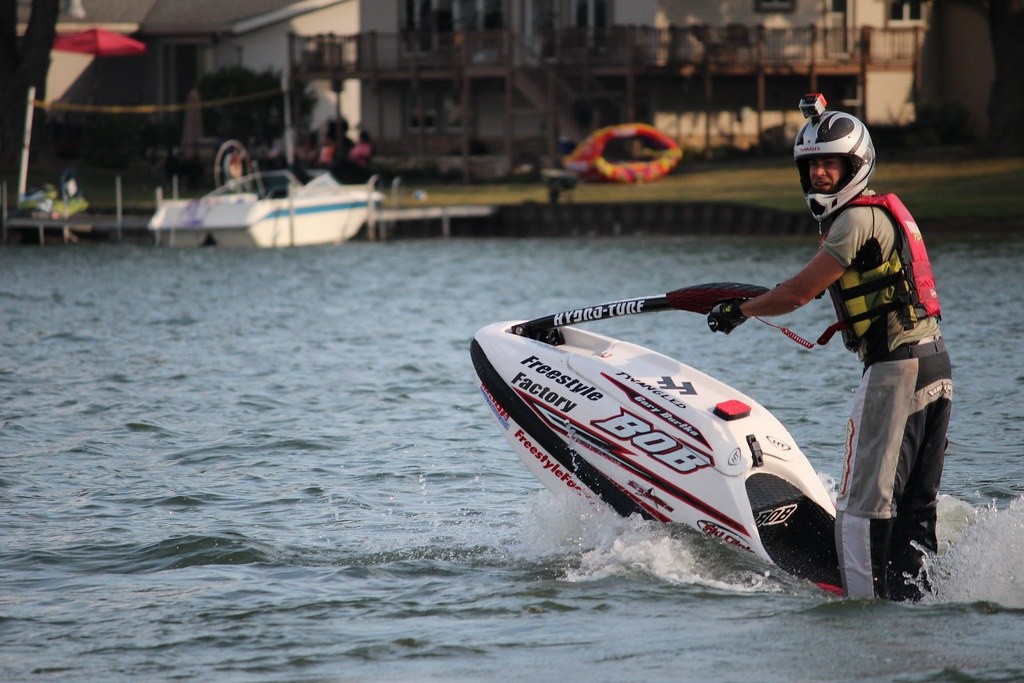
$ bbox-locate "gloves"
[706,303,747,336]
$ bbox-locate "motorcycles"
[469,281,846,599]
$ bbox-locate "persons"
[704,89,956,604]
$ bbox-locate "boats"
[147,167,386,250]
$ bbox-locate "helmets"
[792,92,876,218]
